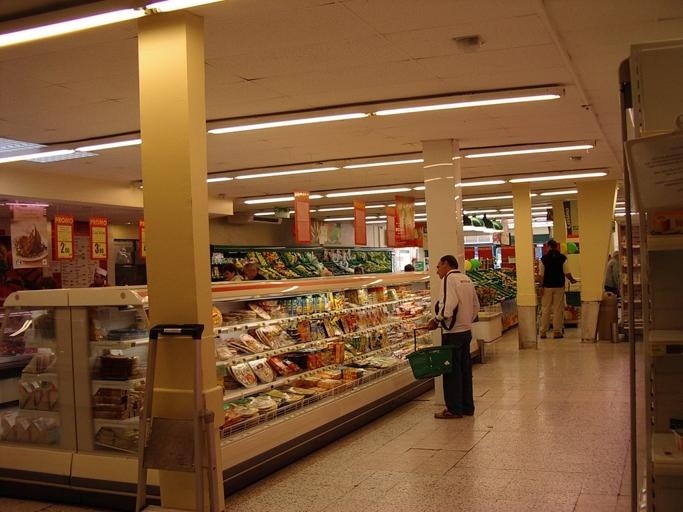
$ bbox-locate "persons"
[540,240,577,339]
[223,264,244,281]
[427,255,481,419]
[87,268,112,287]
[244,264,268,280]
[604,251,620,298]
[404,265,414,272]
[354,267,363,274]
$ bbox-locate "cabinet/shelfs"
[621,35,683,512]
[71,271,434,505]
[466,267,517,334]
[616,216,646,336]
[0,288,77,504]
[210,244,393,283]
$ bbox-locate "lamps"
[463,208,498,214]
[207,110,371,136]
[325,183,411,199]
[254,208,316,217]
[143,0,224,20]
[242,191,322,205]
[137,173,233,190]
[413,176,508,191]
[0,143,74,163]
[414,199,426,206]
[76,132,141,153]
[463,192,538,203]
[539,187,577,197]
[1,7,147,47]
[379,212,427,219]
[509,168,612,184]
[500,204,553,212]
[234,160,341,181]
[462,141,596,160]
[318,203,387,212]
[322,214,378,222]
[373,87,564,117]
[341,154,423,170]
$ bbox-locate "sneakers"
[540,332,547,339]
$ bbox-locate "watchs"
[432,318,439,324]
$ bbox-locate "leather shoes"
[553,332,564,338]
[434,409,474,418]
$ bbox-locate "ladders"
[136,324,219,512]
